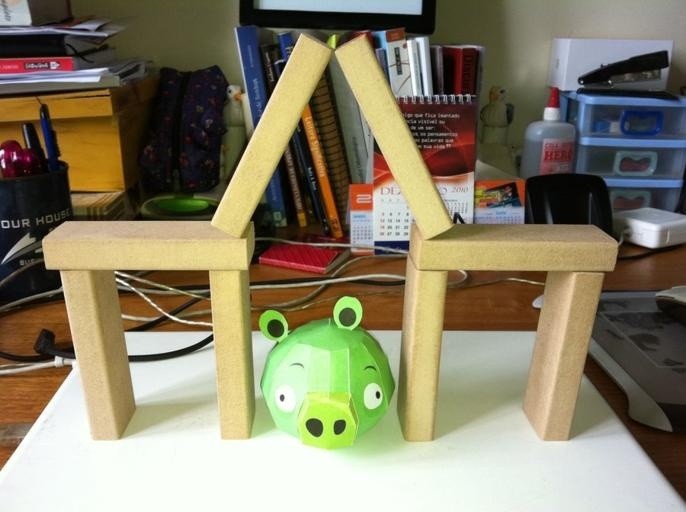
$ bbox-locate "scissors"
[0,140,39,177]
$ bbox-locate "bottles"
[521,87,576,178]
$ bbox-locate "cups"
[0,160,75,250]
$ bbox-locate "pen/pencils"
[22,104,60,173]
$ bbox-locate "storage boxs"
[557,89,686,241]
[0,71,159,281]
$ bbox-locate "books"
[234,26,485,274]
[0,0,149,95]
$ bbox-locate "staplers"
[577,50,679,100]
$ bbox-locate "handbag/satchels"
[138,64,229,195]
